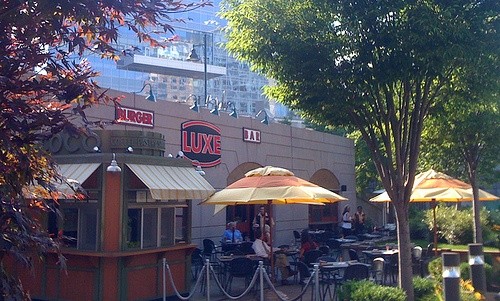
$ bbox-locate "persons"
[341,205,355,236]
[251,231,287,260]
[222,222,244,244]
[298,233,320,261]
[352,206,368,226]
[251,206,276,245]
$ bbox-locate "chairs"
[229,257,254,293]
[347,248,368,263]
[203,238,219,262]
[196,252,225,293]
[369,258,387,289]
[297,259,328,301]
[335,264,369,295]
[412,246,424,277]
[293,229,302,247]
[304,248,328,285]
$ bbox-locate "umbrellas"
[196,165,349,285]
[366,167,500,260]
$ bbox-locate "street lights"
[186,34,207,105]
